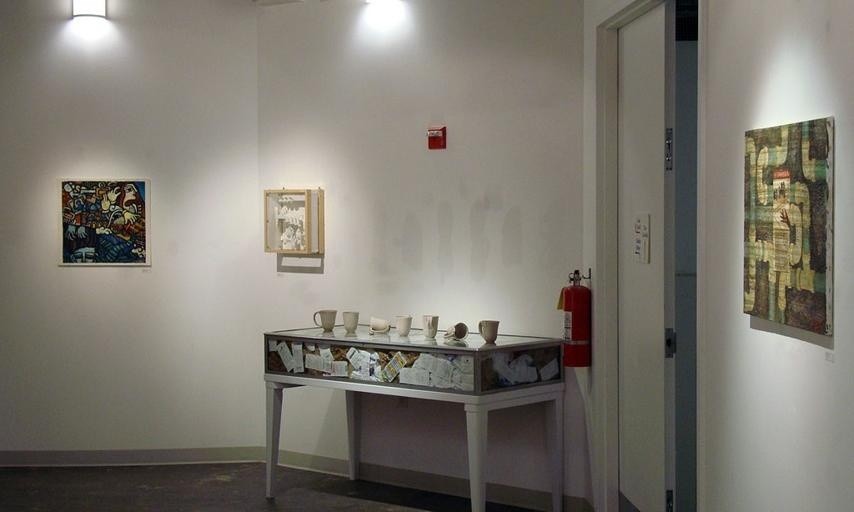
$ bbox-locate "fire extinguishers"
[556,268,593,368]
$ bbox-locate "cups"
[478,319,501,345]
[444,322,469,341]
[313,309,337,332]
[395,315,413,337]
[369,317,392,335]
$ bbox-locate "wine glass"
[342,312,359,337]
[421,314,439,341]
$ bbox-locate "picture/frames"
[59,177,151,266]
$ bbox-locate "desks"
[261,323,566,512]
